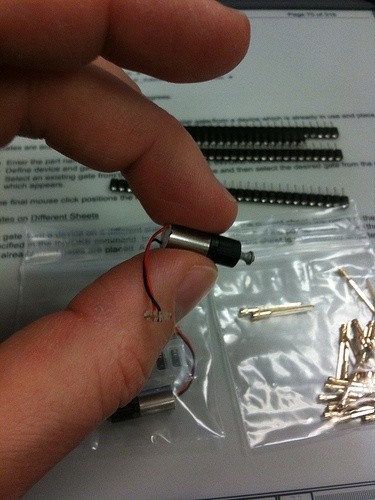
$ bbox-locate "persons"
[0,1,250,500]
